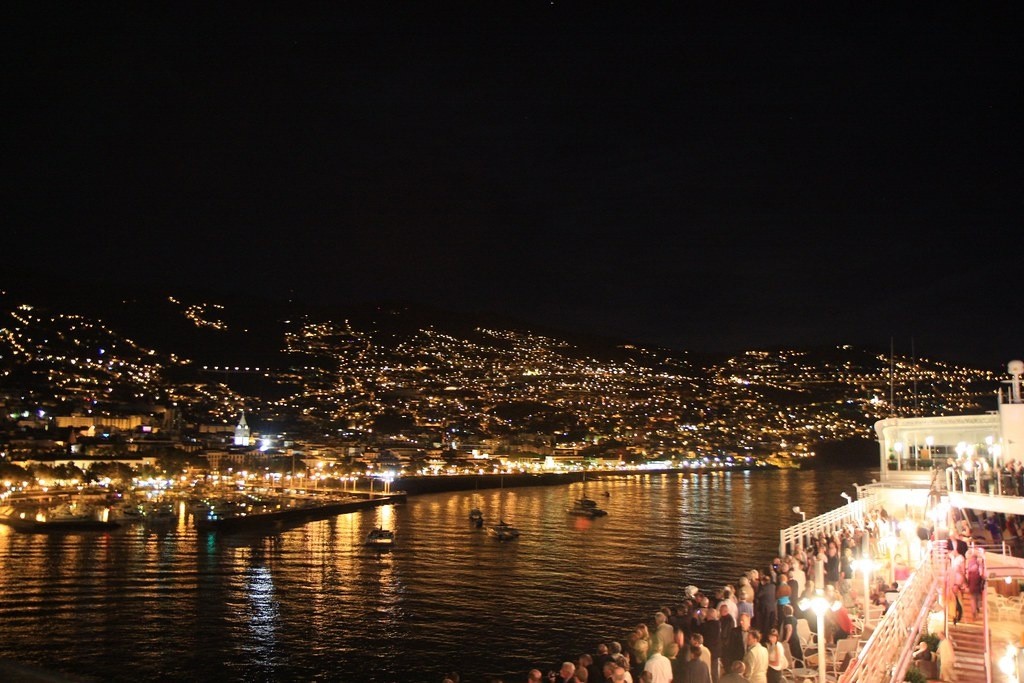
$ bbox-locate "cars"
[265,449,285,454]
[105,491,124,502]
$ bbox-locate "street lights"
[849,529,885,629]
[898,516,918,570]
[894,442,903,471]
[799,559,842,683]
[925,436,934,470]
[879,530,902,585]
[1,467,395,508]
[956,436,1004,493]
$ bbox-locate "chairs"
[988,587,1024,622]
[784,589,900,683]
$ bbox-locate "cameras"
[550,672,559,678]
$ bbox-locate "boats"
[365,529,394,547]
[486,521,521,540]
[469,508,483,521]
[564,498,608,517]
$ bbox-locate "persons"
[782,605,804,667]
[743,631,769,683]
[645,638,674,683]
[912,441,1024,625]
[684,645,710,683]
[934,632,959,682]
[527,506,907,683]
[730,612,766,660]
[655,612,674,654]
[913,641,932,663]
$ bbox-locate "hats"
[736,572,752,580]
